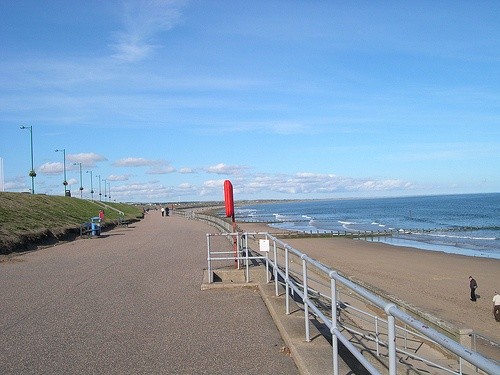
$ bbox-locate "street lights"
[19,125,37,194]
[54,148,68,190]
[73,162,111,203]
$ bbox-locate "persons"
[161,207,165,216]
[469,276,478,301]
[492,291,500,322]
[165,206,169,216]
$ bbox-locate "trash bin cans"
[92,216,101,235]
[65,189,71,197]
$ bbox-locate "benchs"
[117,217,129,229]
[79,222,102,238]
